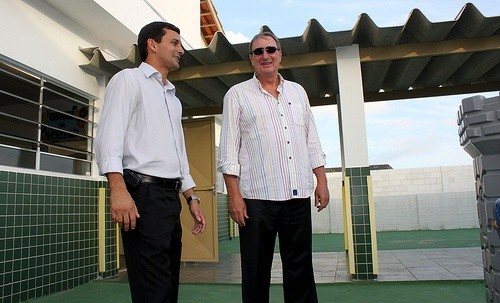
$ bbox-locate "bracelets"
[186,194,201,205]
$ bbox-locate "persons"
[217,32,332,303]
[94,22,206,303]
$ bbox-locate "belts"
[122,169,182,192]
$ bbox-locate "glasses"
[249,46,282,55]
